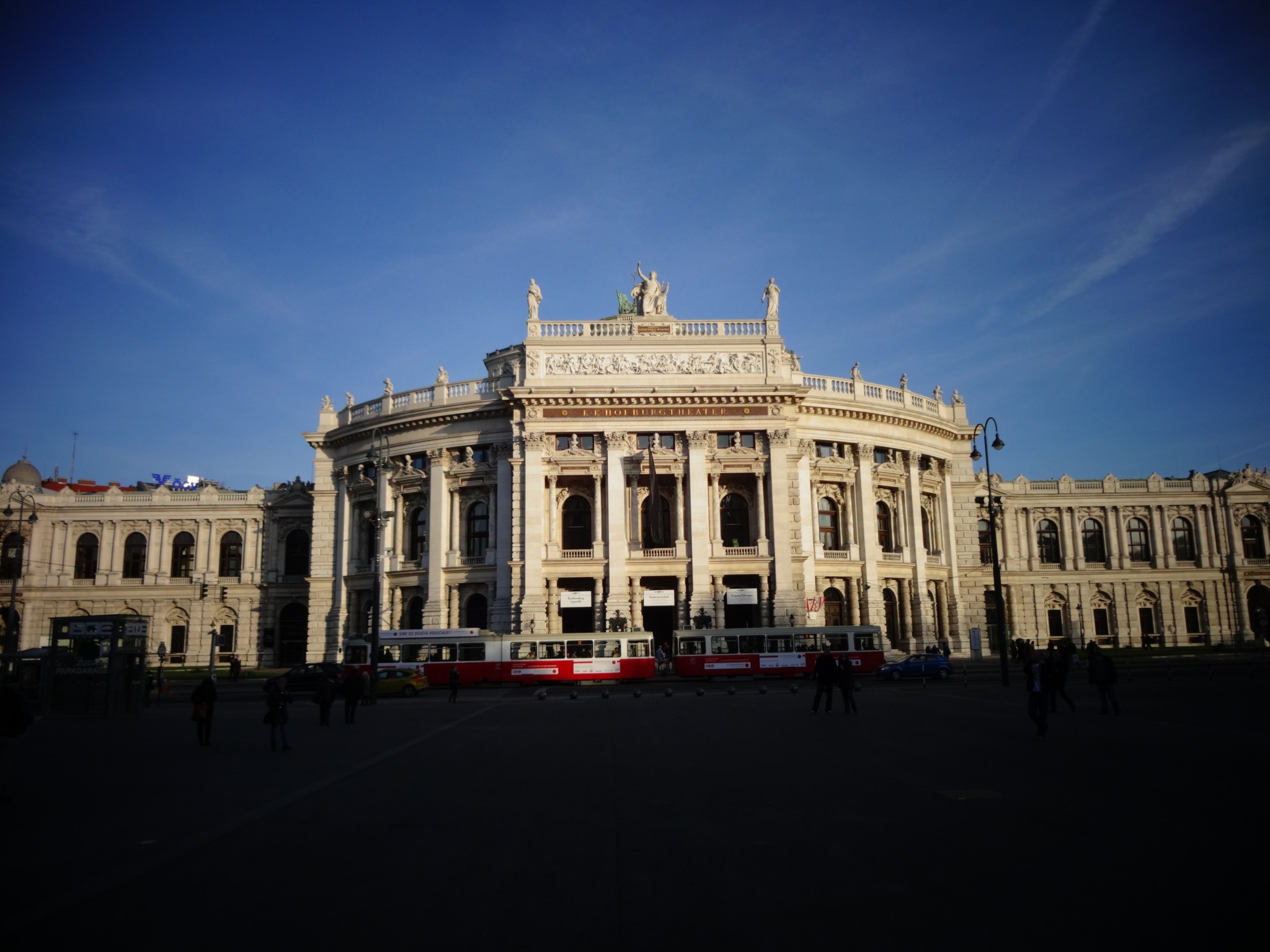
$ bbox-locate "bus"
[674,626,885,677]
[341,629,655,691]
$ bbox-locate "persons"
[321,395,333,409]
[925,633,1153,741]
[358,446,474,476]
[526,644,620,660]
[790,350,965,405]
[425,653,441,662]
[229,653,242,682]
[191,678,217,745]
[527,278,542,320]
[830,442,938,469]
[656,636,875,716]
[436,366,448,383]
[761,278,780,319]
[571,431,741,449]
[266,678,293,751]
[345,391,354,406]
[383,648,394,663]
[316,665,363,725]
[637,261,669,315]
[382,378,393,395]
[447,665,460,703]
[144,670,155,708]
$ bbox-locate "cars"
[875,653,954,681]
[364,667,429,699]
[264,662,359,700]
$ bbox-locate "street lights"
[970,416,1011,684]
[4,489,38,652]
[369,426,393,634]
